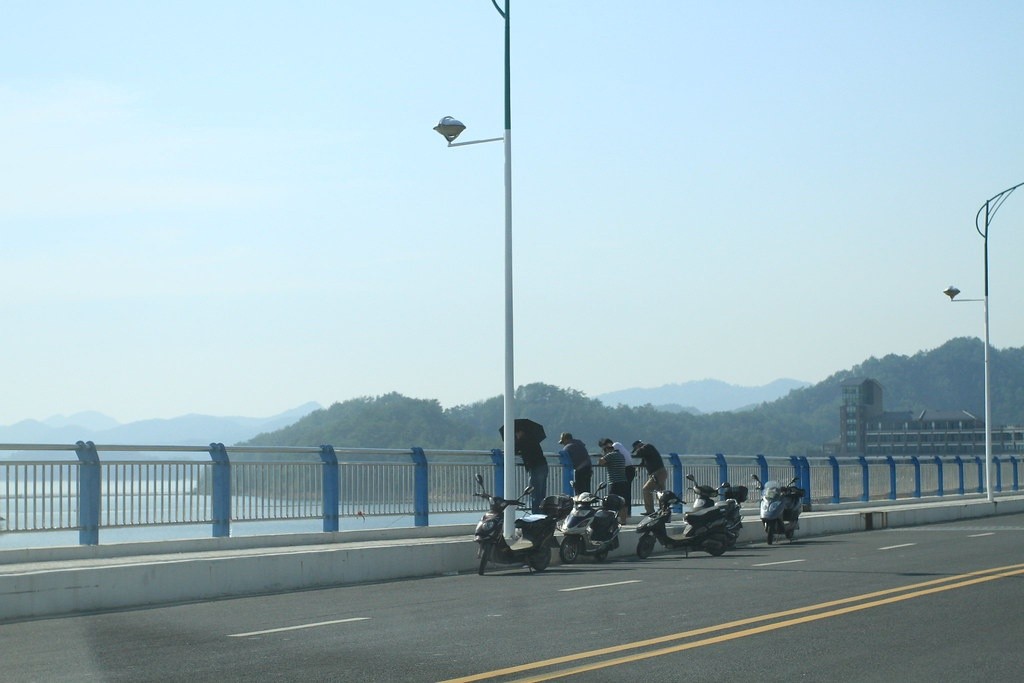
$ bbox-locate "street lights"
[944,184,1024,501]
[433,0,514,538]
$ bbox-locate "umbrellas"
[499,418,547,444]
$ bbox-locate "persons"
[598,438,635,525]
[515,427,549,515]
[630,440,667,515]
[559,432,593,495]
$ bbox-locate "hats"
[558,432,572,444]
[631,440,641,453]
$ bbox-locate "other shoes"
[641,512,651,515]
[619,521,626,525]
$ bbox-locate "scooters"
[636,473,747,561]
[468,472,568,576]
[557,480,627,565]
[754,473,806,544]
